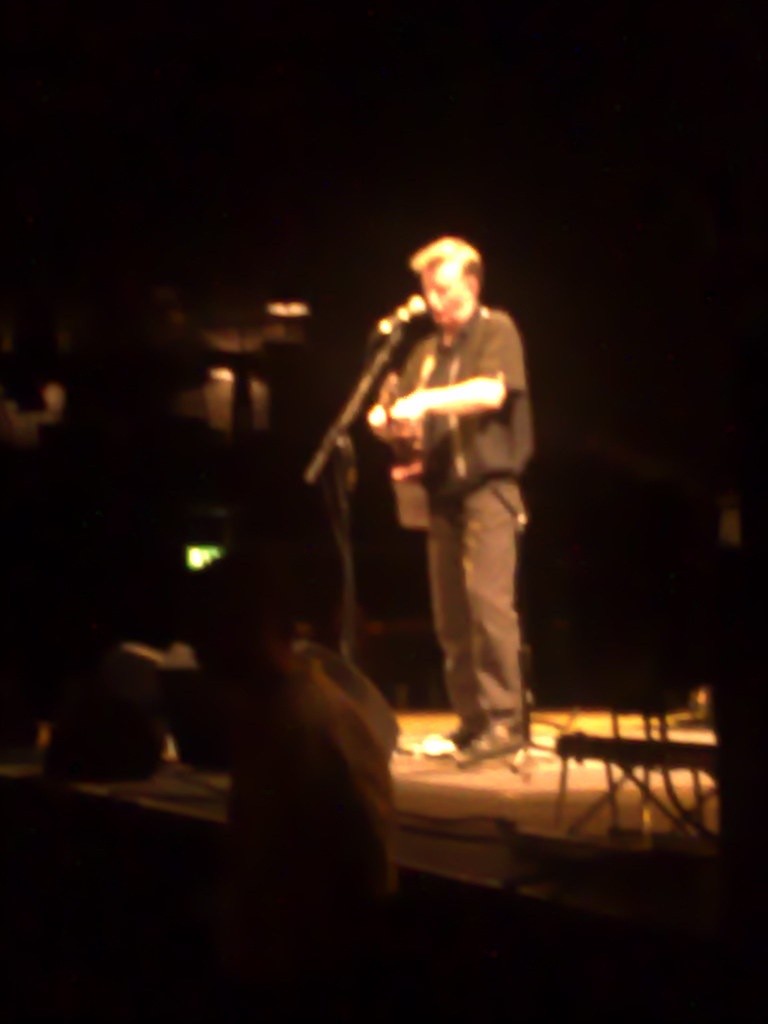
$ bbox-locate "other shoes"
[442,729,472,751]
[455,727,529,765]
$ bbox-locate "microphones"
[372,294,427,338]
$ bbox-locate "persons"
[367,237,532,766]
[0,300,403,1024]
